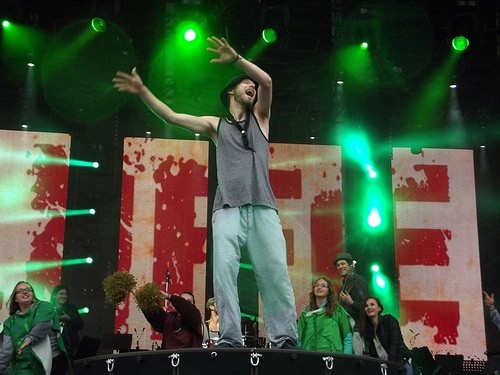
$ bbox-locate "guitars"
[335,259,359,304]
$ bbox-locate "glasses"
[314,282,328,288]
[17,288,30,293]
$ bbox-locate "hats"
[334,253,352,265]
[219,75,259,108]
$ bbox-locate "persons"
[112,36,302,351]
[483,291,500,329]
[0,281,85,375]
[129,253,413,375]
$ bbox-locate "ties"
[227,110,256,152]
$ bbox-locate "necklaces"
[227,107,256,153]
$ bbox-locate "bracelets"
[232,54,239,64]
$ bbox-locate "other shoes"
[271,342,298,350]
[215,343,231,348]
